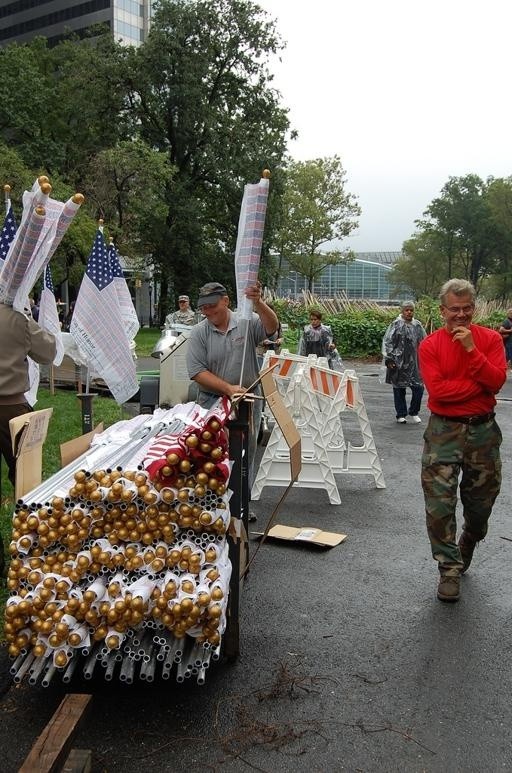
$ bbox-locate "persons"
[164,294,195,326]
[0,303,57,577]
[297,310,337,371]
[185,281,279,522]
[256,303,284,356]
[382,300,428,425]
[29,298,76,333]
[499,308,512,368]
[417,277,507,603]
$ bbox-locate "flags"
[38,264,65,366]
[67,217,141,408]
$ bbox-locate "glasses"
[441,304,471,312]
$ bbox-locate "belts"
[447,413,496,426]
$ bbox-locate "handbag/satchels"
[496,325,509,338]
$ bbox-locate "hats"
[197,282,228,307]
[177,295,189,301]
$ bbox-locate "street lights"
[147,285,153,328]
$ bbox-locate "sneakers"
[397,417,408,424]
[458,530,477,574]
[437,576,462,602]
[248,508,257,523]
[413,415,422,423]
